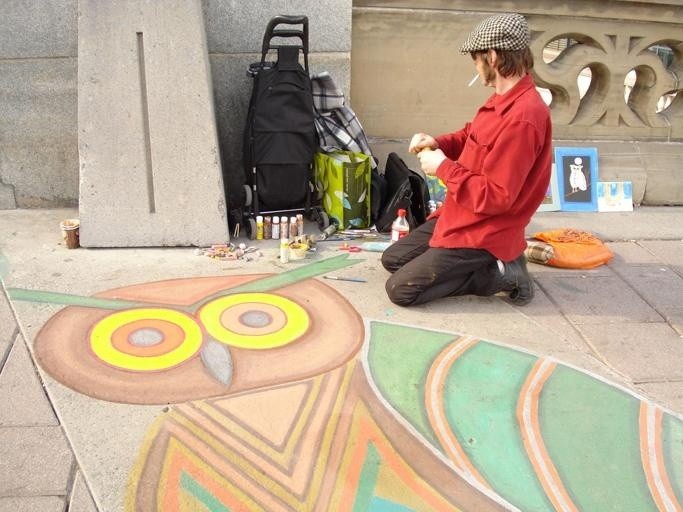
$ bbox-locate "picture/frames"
[553,147,599,212]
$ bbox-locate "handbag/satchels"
[315,149,371,230]
[376,153,428,233]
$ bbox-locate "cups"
[59,218,79,250]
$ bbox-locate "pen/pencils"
[339,228,377,238]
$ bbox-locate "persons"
[381,13,553,306]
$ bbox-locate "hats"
[459,13,530,55]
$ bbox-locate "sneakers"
[501,253,534,306]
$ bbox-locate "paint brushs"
[316,238,354,242]
[323,275,367,282]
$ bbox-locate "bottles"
[391,209,409,242]
[255,214,306,263]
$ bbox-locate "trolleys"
[242,15,330,240]
